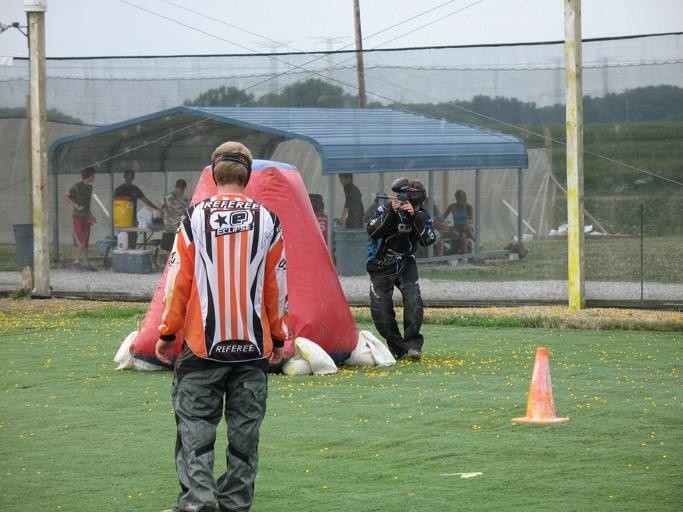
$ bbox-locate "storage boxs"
[111,249,152,273]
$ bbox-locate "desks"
[114,227,163,270]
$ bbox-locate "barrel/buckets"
[335,227,370,277]
[13,223,34,271]
[112,196,135,228]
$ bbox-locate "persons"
[64,167,97,272]
[366,178,436,362]
[155,140,288,512]
[309,173,473,256]
[114,169,160,249]
[159,179,192,251]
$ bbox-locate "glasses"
[408,195,422,206]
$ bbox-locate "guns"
[376,178,426,224]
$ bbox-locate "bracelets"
[273,340,285,348]
[159,335,175,342]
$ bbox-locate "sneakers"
[69,261,98,273]
[390,347,421,361]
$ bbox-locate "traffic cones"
[510,347,571,425]
